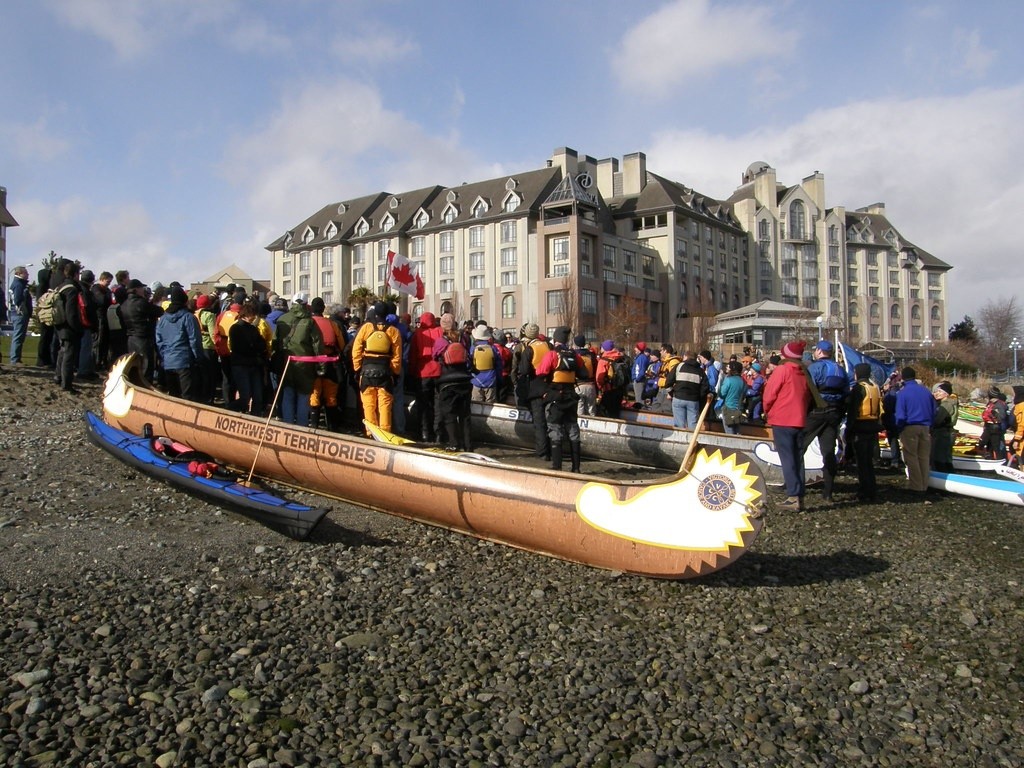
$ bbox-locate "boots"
[571,440,580,472]
[550,440,562,470]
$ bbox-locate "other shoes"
[10,358,99,394]
[774,452,933,513]
[964,444,1024,470]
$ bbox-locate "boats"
[343,383,847,489]
[101,352,769,582]
[905,466,1024,505]
[877,430,1018,471]
[83,406,334,540]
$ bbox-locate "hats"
[700,350,711,361]
[751,363,762,373]
[602,339,615,351]
[811,341,833,352]
[939,382,953,396]
[35,254,592,353]
[781,341,806,360]
[770,355,781,366]
[730,362,743,373]
[636,342,646,352]
[988,386,1000,398]
[902,366,915,381]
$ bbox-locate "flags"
[838,339,896,390]
[384,250,425,300]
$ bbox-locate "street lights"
[1008,337,1022,376]
[919,335,934,360]
[817,316,823,341]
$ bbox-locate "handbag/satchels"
[724,409,742,426]
[269,349,296,380]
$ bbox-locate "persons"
[34,257,765,475]
[760,338,958,515]
[964,383,1024,472]
[6,266,33,365]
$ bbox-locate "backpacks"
[602,358,629,389]
[38,284,76,326]
